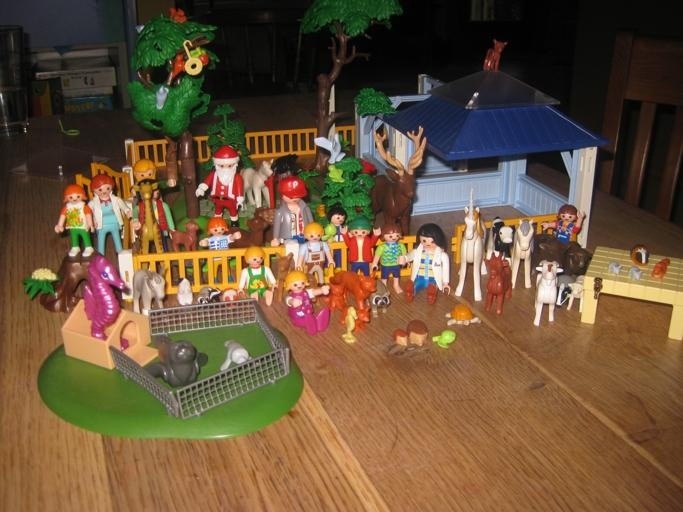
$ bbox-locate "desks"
[0,84,682,512]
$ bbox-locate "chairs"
[594,30,682,228]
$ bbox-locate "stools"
[577,244,681,341]
